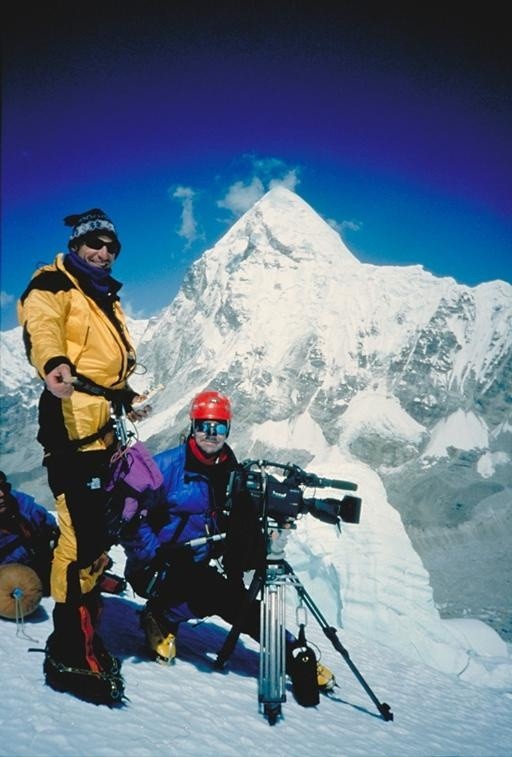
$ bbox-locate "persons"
[121,391,335,691]
[17,208,153,703]
[0,471,126,594]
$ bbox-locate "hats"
[63,206,121,249]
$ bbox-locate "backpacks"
[99,436,169,547]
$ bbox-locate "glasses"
[77,233,120,255]
[190,419,230,438]
[0,481,12,493]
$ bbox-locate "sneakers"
[136,601,181,667]
[316,657,338,693]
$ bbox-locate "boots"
[38,598,131,710]
[97,571,127,595]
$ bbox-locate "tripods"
[212,556,395,725]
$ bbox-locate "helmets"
[189,389,233,422]
